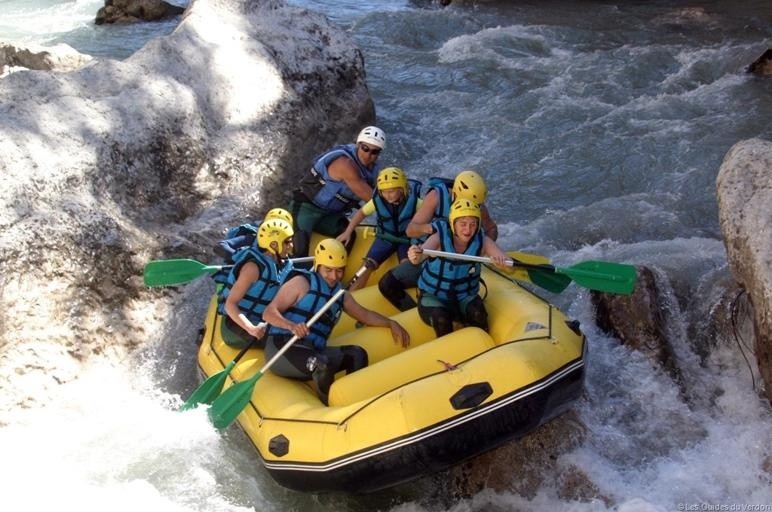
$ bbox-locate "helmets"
[263,207,294,228]
[313,238,348,276]
[376,166,410,200]
[356,125,388,153]
[448,198,482,237]
[256,217,295,257]
[451,170,488,207]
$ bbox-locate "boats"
[195,221,588,494]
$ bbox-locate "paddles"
[368,230,636,297]
[207,263,368,431]
[179,319,270,413]
[143,257,320,286]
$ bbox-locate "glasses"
[359,141,382,155]
